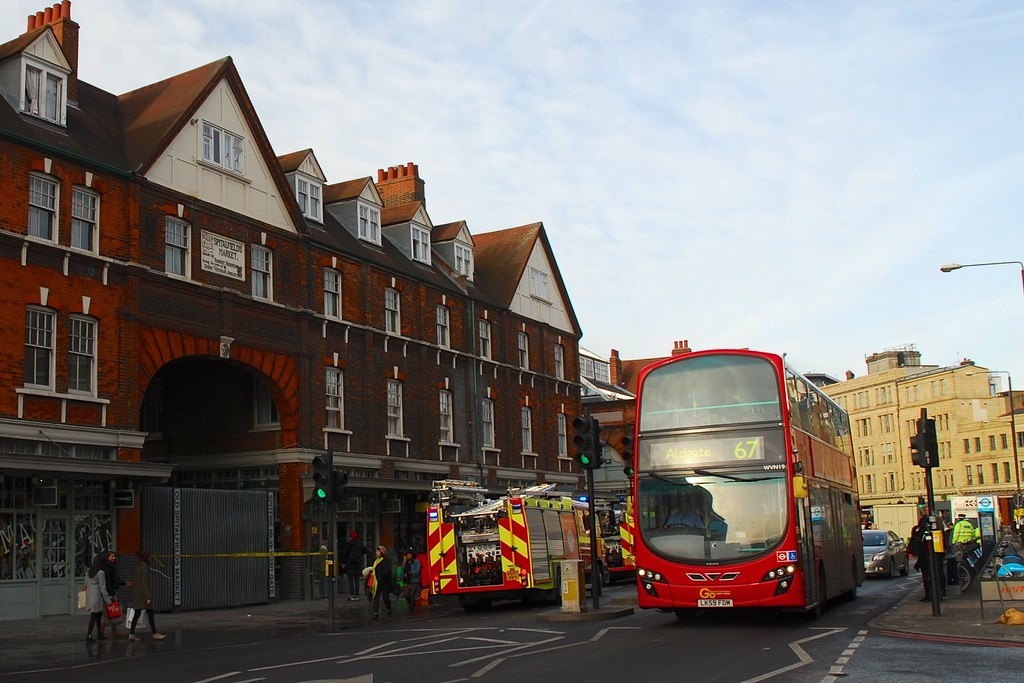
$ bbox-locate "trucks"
[873,504,920,543]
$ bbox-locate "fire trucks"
[598,498,637,576]
[428,478,617,612]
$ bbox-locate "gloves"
[147,600,152,606]
[125,581,132,588]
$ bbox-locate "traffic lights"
[909,434,926,467]
[593,419,606,468]
[573,416,598,469]
[621,436,634,479]
[918,497,923,508]
[312,455,332,506]
[333,471,349,500]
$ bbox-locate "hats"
[379,545,387,556]
[406,550,416,558]
[350,531,358,539]
[136,551,149,562]
[322,540,328,545]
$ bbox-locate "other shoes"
[153,633,166,640]
[86,634,95,641]
[383,608,391,612]
[941,592,947,599]
[371,609,378,615]
[919,597,930,602]
[355,596,360,600]
[98,632,105,640]
[349,593,355,601]
[129,634,140,642]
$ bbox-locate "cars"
[862,529,909,578]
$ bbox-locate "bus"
[635,349,866,618]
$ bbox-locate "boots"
[101,624,110,639]
[111,627,125,638]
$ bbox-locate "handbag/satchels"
[128,608,146,624]
[126,608,147,630]
[105,601,121,618]
[366,574,373,588]
[344,560,364,575]
[78,590,87,609]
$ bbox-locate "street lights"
[940,261,1024,290]
[966,370,1024,520]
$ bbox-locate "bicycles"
[943,536,1024,592]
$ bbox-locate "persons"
[128,552,167,641]
[948,523,953,528]
[401,550,423,616]
[919,506,929,525]
[319,541,330,600]
[947,514,974,585]
[864,523,878,530]
[85,551,132,642]
[664,497,704,529]
[344,531,372,601]
[910,517,951,602]
[371,545,392,620]
